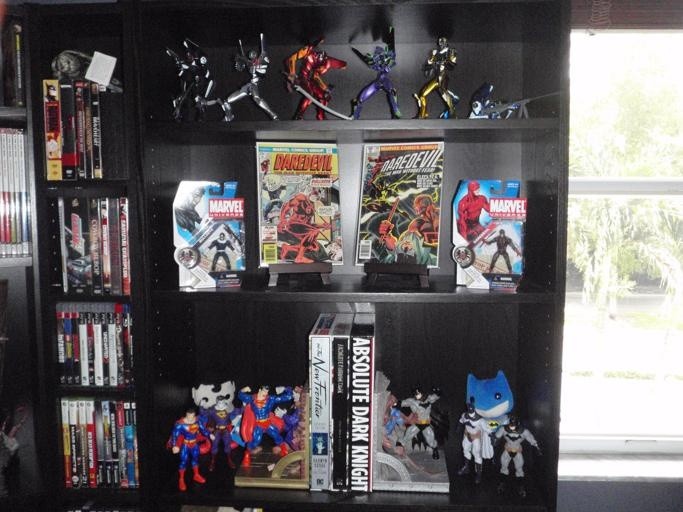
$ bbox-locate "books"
[93,303,104,386]
[351,312,375,493]
[452,179,527,289]
[61,396,71,487]
[79,398,88,490]
[89,198,102,295]
[56,301,65,384]
[111,400,120,488]
[256,142,344,267]
[78,302,89,386]
[43,80,63,181]
[115,303,125,388]
[308,313,334,491]
[102,399,113,489]
[124,401,134,487]
[132,401,140,487]
[19,130,29,257]
[108,302,118,387]
[356,142,444,269]
[86,397,99,488]
[59,79,78,182]
[116,401,127,488]
[47,197,68,294]
[83,82,93,179]
[74,81,86,180]
[91,81,103,180]
[64,301,73,385]
[101,198,111,296]
[331,313,354,491]
[172,180,246,292]
[72,302,81,385]
[86,302,95,387]
[128,303,135,385]
[8,128,16,243]
[9,14,26,107]
[100,303,109,387]
[124,303,130,385]
[2,128,11,244]
[95,398,106,487]
[69,398,80,487]
[63,198,92,296]
[119,197,131,296]
[13,129,22,243]
[0,189,6,243]
[108,197,123,297]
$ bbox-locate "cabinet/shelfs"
[28,0,573,512]
[0,0,47,512]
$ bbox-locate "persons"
[495,414,541,499]
[237,382,293,467]
[208,233,237,271]
[486,230,522,274]
[260,176,286,219]
[409,35,460,120]
[278,191,331,263]
[273,383,308,466]
[382,394,409,456]
[395,383,443,461]
[379,194,440,253]
[158,35,217,120]
[454,403,494,486]
[218,33,282,122]
[381,234,438,267]
[202,396,240,471]
[457,180,490,241]
[283,34,350,122]
[348,42,401,119]
[167,408,215,490]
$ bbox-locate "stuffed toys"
[465,370,513,445]
